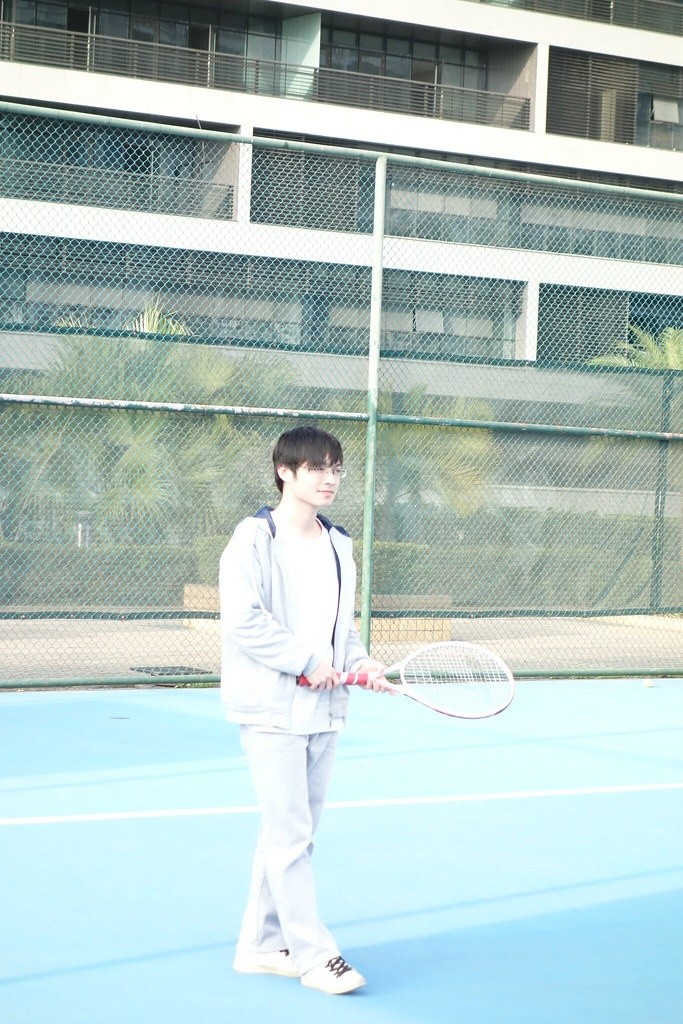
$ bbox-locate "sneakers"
[301,956,367,994]
[233,948,299,978]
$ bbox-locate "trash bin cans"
[77,511,92,547]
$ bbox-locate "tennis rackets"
[296,639,518,719]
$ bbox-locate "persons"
[219,425,395,994]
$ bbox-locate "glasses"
[296,462,347,478]
